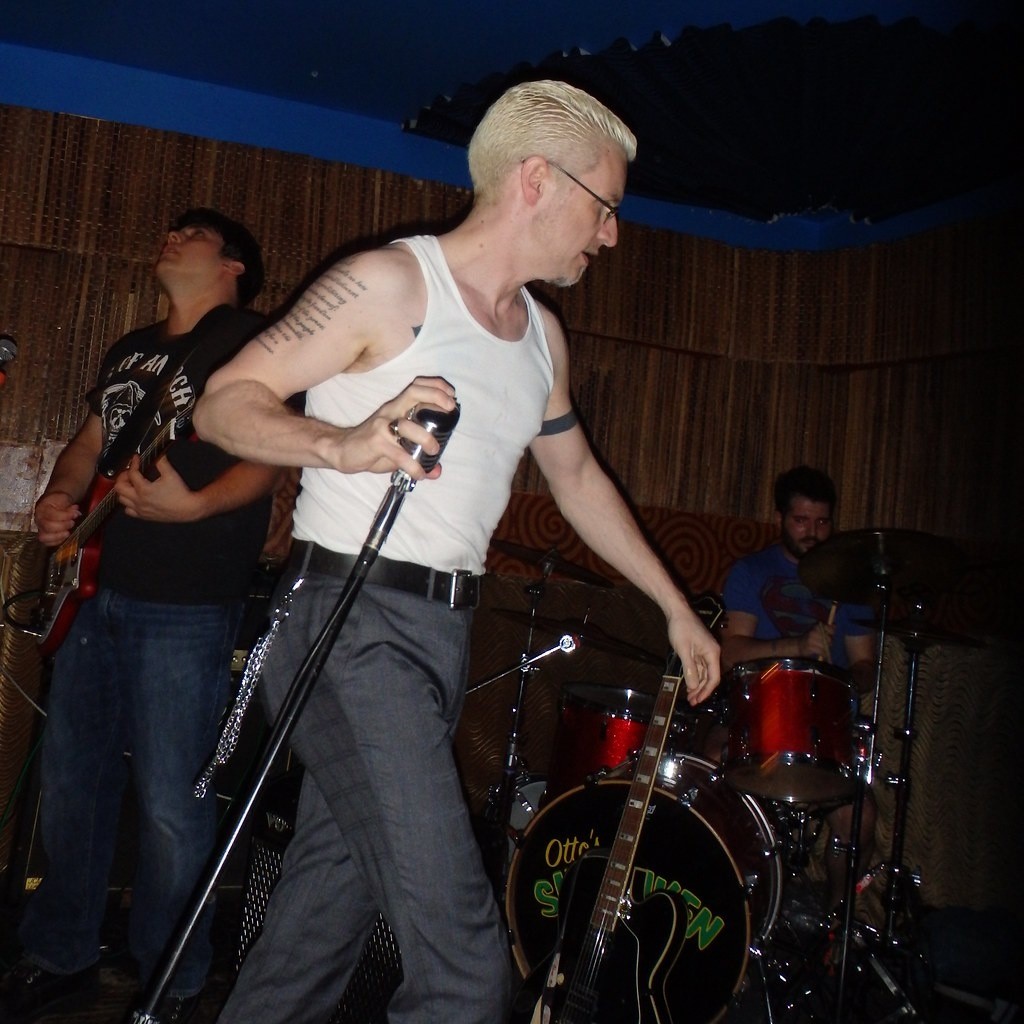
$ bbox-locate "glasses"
[522,155,620,225]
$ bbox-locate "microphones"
[0,338,17,367]
[362,400,462,567]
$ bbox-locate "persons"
[0,208,300,1024]
[722,464,882,977]
[189,81,721,1024]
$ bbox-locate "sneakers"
[0,959,99,1024]
[122,988,203,1024]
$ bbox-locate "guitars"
[530,587,730,1024]
[11,399,204,668]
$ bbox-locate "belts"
[287,540,489,610]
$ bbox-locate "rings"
[389,417,401,436]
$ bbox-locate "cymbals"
[799,528,991,608]
[488,607,657,668]
[488,536,615,590]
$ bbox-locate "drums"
[498,745,785,1024]
[556,682,691,778]
[716,654,863,809]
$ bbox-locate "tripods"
[769,568,935,1024]
[475,565,556,900]
